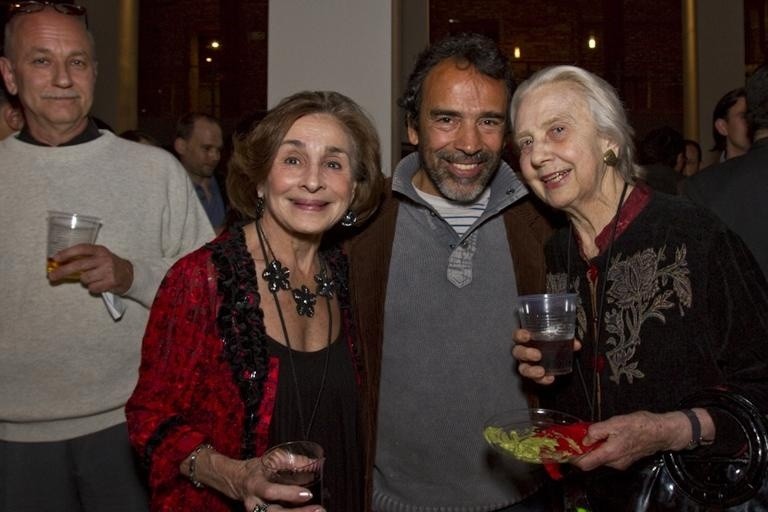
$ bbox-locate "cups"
[261,440,325,509]
[512,291,578,378]
[45,209,102,281]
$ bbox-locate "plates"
[482,406,596,465]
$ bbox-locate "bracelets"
[189,445,216,488]
[682,407,703,450]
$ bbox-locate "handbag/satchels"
[625,385,768,512]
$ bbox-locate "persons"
[338,34,556,512]
[123,91,385,512]
[508,62,768,512]
[119,130,160,146]
[638,64,768,279]
[170,111,226,236]
[0,1,218,512]
[0,90,25,140]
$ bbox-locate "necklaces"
[257,224,335,318]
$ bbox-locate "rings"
[252,503,270,512]
